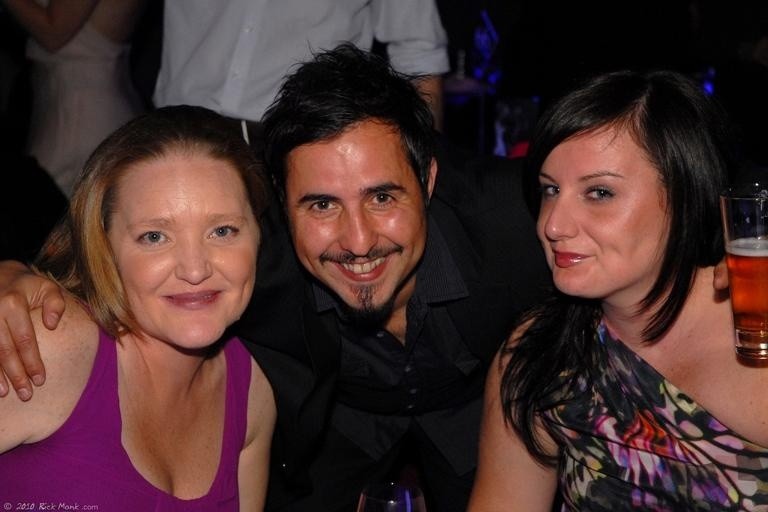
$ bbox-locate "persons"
[0,102,279,511]
[149,0,458,135]
[0,41,767,510]
[1,0,153,279]
[458,63,768,512]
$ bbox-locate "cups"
[718,188,768,368]
[354,479,426,512]
